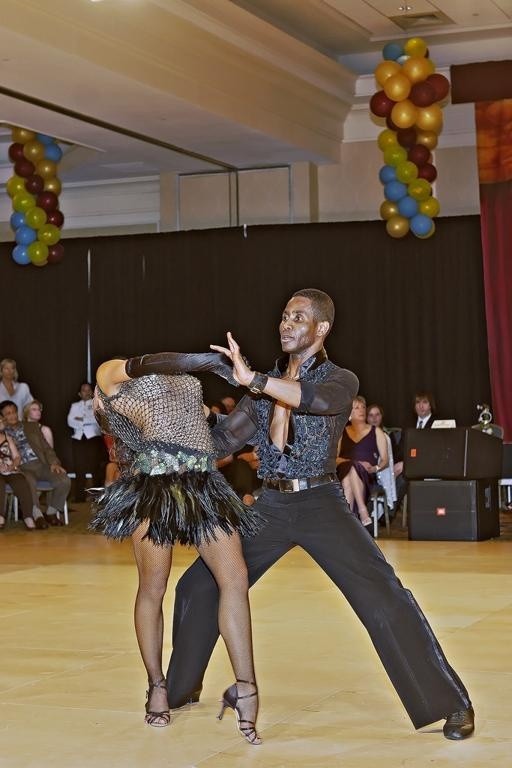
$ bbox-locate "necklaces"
[285,370,300,379]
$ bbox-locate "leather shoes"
[23,517,36,531]
[443,706,474,741]
[34,515,48,529]
[0,515,6,529]
[43,512,65,527]
[146,686,202,710]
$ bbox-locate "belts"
[261,473,340,494]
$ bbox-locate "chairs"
[470,422,504,511]
[2,483,22,524]
[340,420,407,539]
[36,467,70,529]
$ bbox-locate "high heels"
[144,678,170,728]
[218,677,263,745]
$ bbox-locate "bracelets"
[248,371,269,396]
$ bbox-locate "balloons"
[370,37,451,239]
[5,123,65,266]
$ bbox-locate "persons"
[68,382,121,504]
[336,390,441,536]
[91,350,267,743]
[1,359,71,530]
[210,397,263,507]
[165,288,475,739]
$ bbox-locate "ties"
[418,420,423,429]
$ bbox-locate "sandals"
[359,506,372,526]
